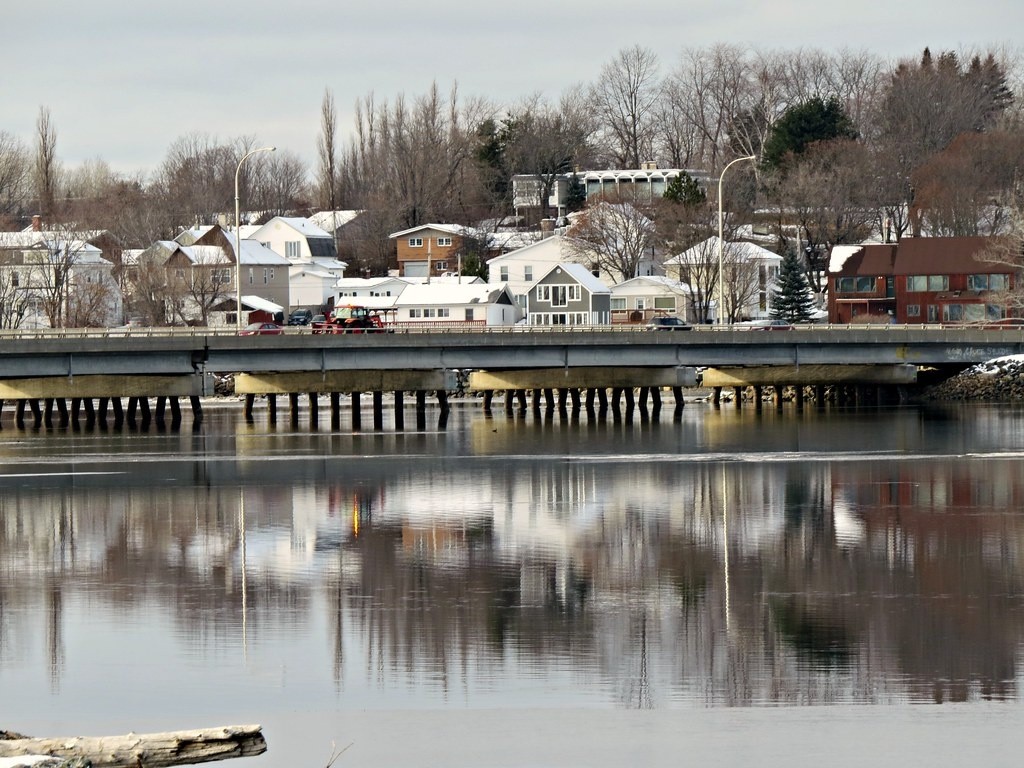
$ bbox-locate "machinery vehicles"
[308,302,382,336]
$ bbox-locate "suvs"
[287,309,313,326]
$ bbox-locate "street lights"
[718,154,759,325]
[235,147,276,334]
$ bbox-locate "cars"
[645,316,692,332]
[128,315,147,328]
[237,323,284,337]
[749,320,793,334]
[310,315,326,327]
